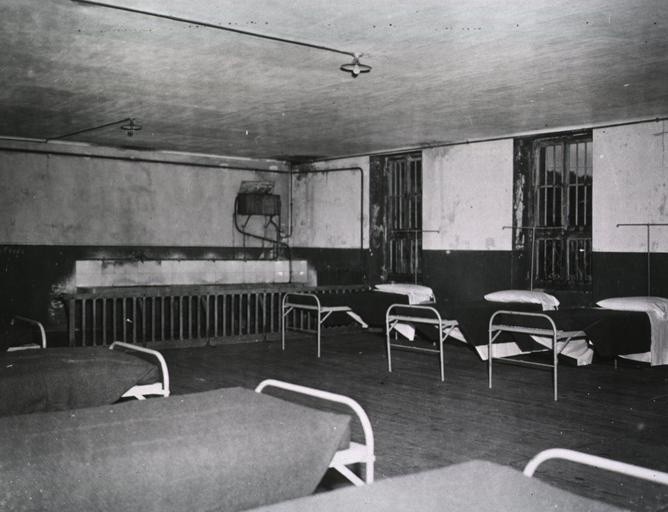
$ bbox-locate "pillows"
[597,297,668,312]
[484,290,560,307]
[375,284,432,297]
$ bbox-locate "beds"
[254,447,668,511]
[1,378,374,507]
[2,341,169,415]
[488,297,667,401]
[386,290,581,383]
[1,314,47,354]
[282,283,437,358]
